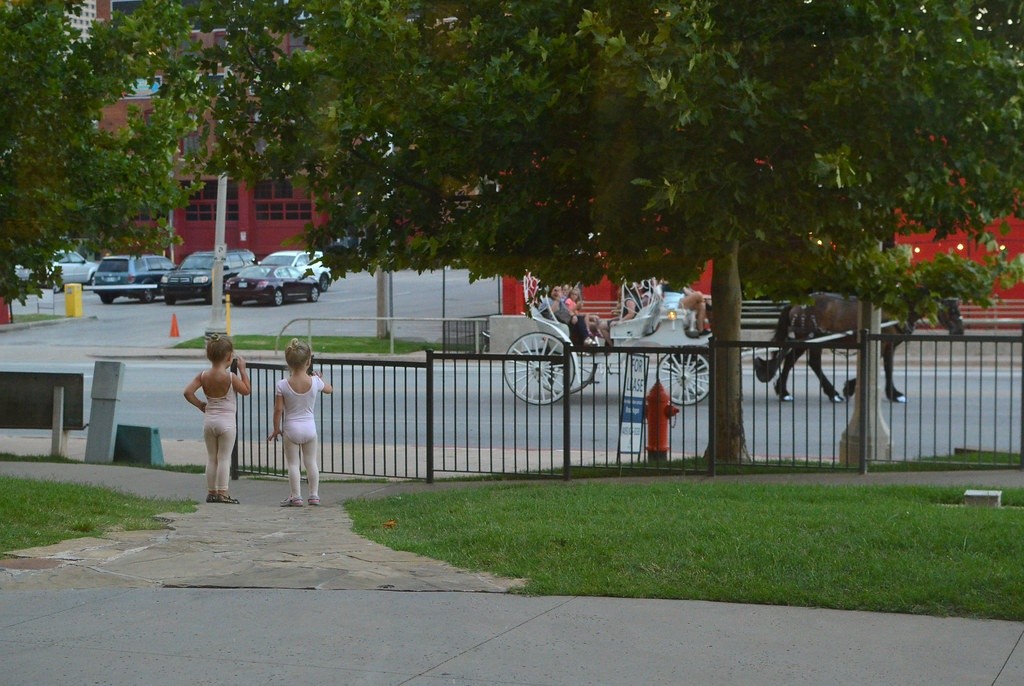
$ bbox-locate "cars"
[225,264,320,306]
[35,250,99,293]
[262,249,333,292]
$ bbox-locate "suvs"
[93,255,178,304]
[160,251,246,306]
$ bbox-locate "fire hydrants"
[642,381,680,463]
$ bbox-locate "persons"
[265,337,332,507]
[539,274,711,357]
[184,333,250,504]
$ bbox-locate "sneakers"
[307,495,320,506]
[206,494,218,502]
[278,495,303,507]
[217,494,240,504]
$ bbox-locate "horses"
[754,291,966,402]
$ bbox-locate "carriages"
[501,259,965,408]
